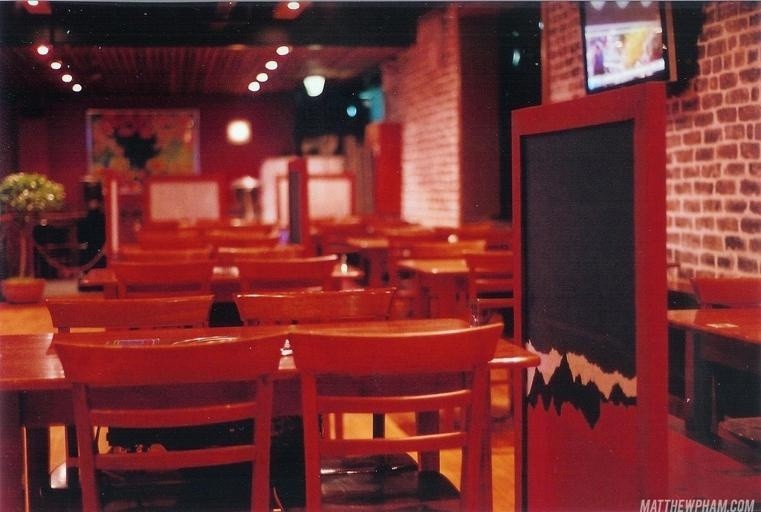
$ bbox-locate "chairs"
[41,218,761,512]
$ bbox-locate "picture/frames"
[84,107,202,176]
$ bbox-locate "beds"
[0,315,542,512]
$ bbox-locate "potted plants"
[0,171,67,304]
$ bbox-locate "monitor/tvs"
[578,0,678,96]
